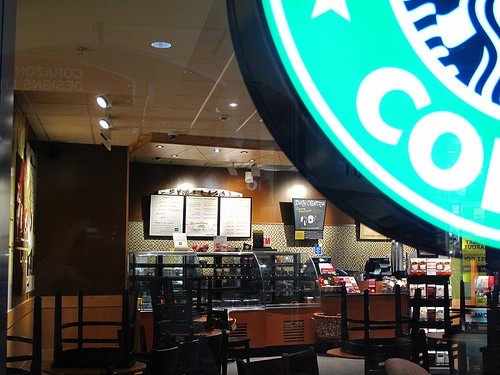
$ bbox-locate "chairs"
[0,256,500,375]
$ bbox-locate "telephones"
[336,268,348,276]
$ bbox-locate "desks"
[39,349,146,375]
[172,328,230,375]
[326,348,365,359]
[425,332,488,375]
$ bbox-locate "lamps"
[95,93,113,109]
[226,162,238,176]
[98,116,114,130]
[251,163,261,176]
[99,132,112,151]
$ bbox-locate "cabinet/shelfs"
[190,251,266,349]
[251,251,322,353]
[407,260,456,329]
[129,252,191,350]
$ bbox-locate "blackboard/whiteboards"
[293,198,326,231]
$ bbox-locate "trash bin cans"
[314,312,342,355]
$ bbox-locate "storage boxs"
[313,313,342,340]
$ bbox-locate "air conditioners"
[245,172,254,183]
